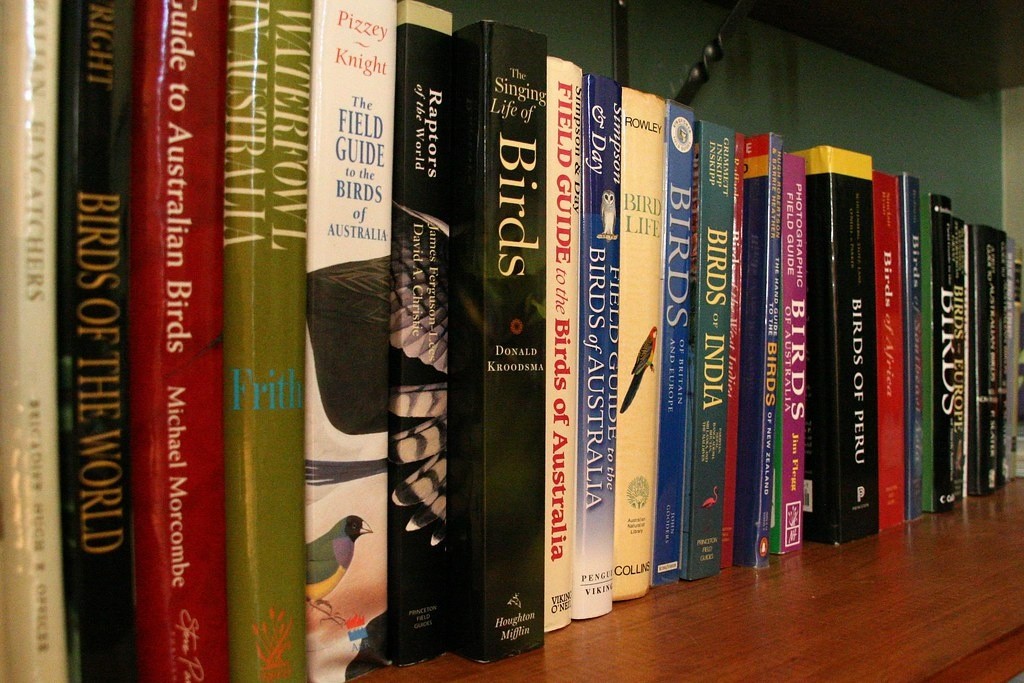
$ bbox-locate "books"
[0,1,1024,683]
